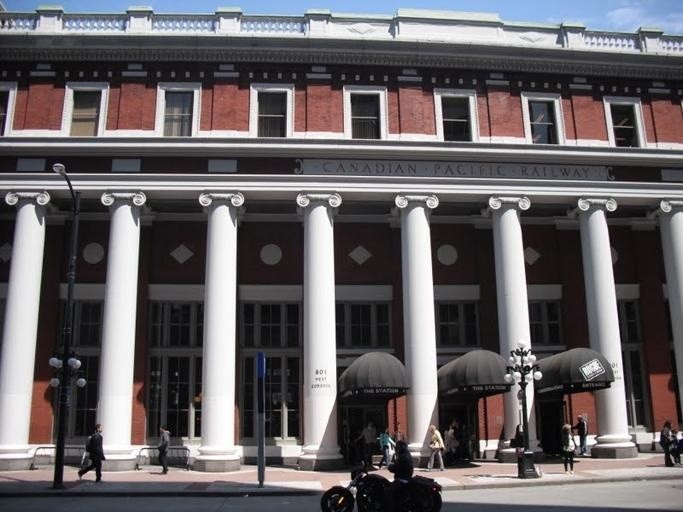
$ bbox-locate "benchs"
[135,447,191,471]
[28,446,86,474]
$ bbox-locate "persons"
[443,416,471,463]
[388,439,411,482]
[659,420,674,467]
[75,422,103,482]
[157,426,170,474]
[553,424,575,474]
[426,424,445,471]
[353,420,376,448]
[571,414,586,455]
[377,424,395,469]
[670,429,682,464]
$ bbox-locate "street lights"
[501,338,543,480]
[42,352,86,491]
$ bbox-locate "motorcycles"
[318,461,445,512]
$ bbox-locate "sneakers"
[78,470,83,479]
[565,470,574,474]
[95,478,106,484]
